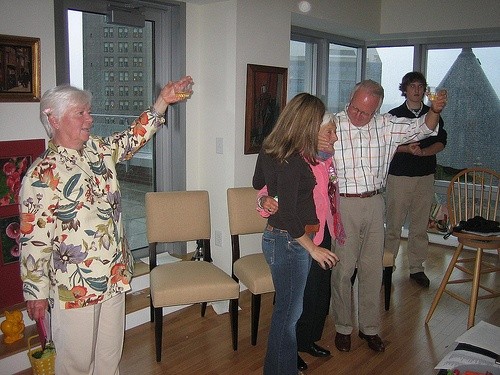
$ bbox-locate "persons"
[318,78,448,352]
[385,71,447,286]
[254,93,338,375]
[18,75,193,375]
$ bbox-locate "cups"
[317,256,340,271]
[426,80,444,100]
[173,80,192,100]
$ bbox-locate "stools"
[425,167,500,331]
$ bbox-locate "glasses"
[348,103,377,118]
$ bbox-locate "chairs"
[145,190,240,362]
[227,188,276,346]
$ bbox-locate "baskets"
[27,335,56,375]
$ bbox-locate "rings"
[268,207,272,213]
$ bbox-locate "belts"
[304,223,320,233]
[338,188,387,198]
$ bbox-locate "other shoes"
[410,271,430,286]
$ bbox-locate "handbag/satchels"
[443,215,500,239]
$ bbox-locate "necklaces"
[47,145,99,189]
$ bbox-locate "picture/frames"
[244,64,288,154]
[0,34,41,102]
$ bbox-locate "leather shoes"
[297,353,308,371]
[358,329,385,352]
[335,331,352,351]
[298,342,331,358]
